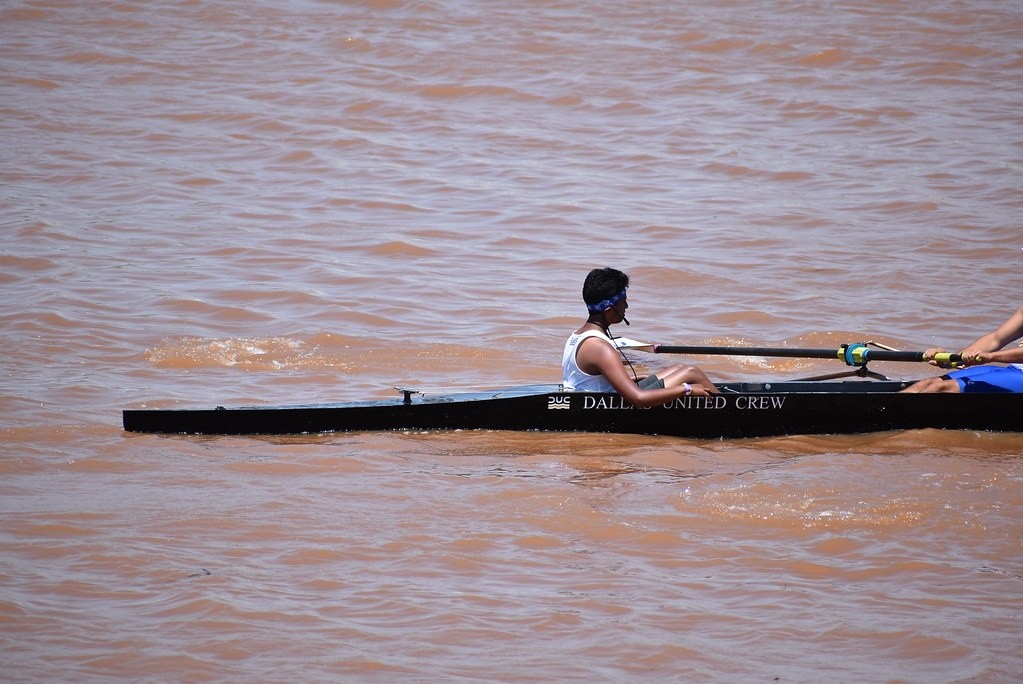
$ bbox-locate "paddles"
[610,334,983,367]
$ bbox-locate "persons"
[898,305,1023,394]
[559,267,722,408]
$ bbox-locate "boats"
[122,368,1023,438]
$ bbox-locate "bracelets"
[681,382,692,395]
